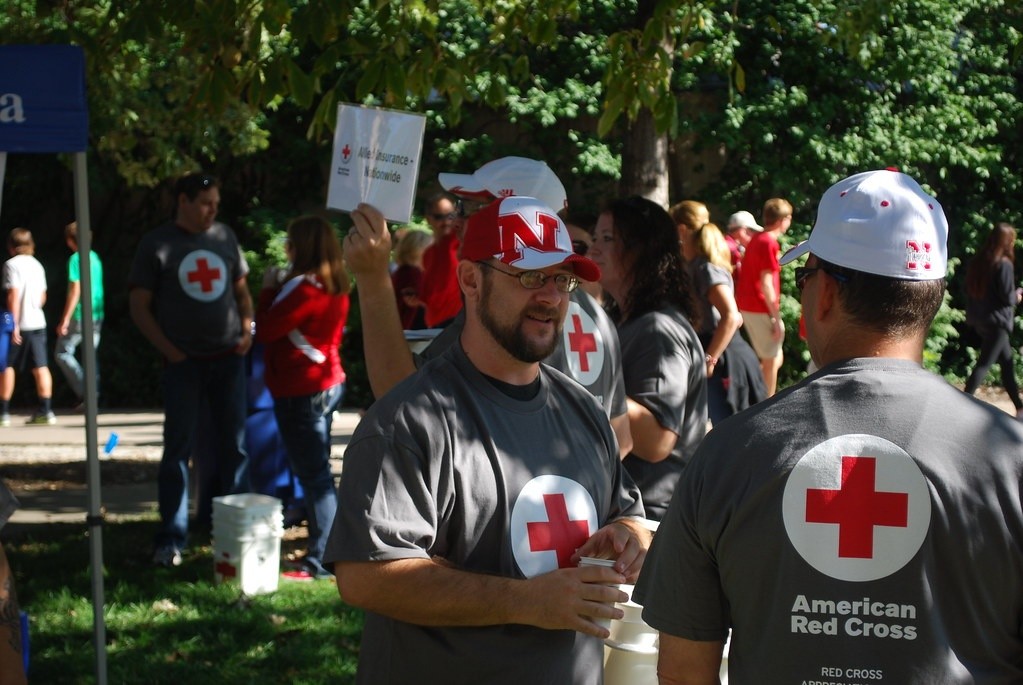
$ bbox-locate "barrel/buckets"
[211,493,284,596]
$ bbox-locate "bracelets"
[705,352,719,365]
[241,317,256,336]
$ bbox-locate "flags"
[0,41,91,154]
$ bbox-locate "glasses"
[451,197,471,218]
[794,265,852,292]
[476,260,580,293]
[431,213,456,222]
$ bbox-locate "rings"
[348,226,356,239]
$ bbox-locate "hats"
[728,210,764,234]
[439,155,571,220]
[777,167,950,281]
[459,196,602,284]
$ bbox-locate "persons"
[633,166,1022,685]
[127,177,257,583]
[0,539,30,685]
[595,195,714,523]
[256,213,349,575]
[721,198,803,403]
[0,227,58,423]
[341,153,635,462]
[52,221,105,414]
[668,199,768,425]
[322,193,661,685]
[965,221,1022,410]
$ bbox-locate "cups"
[579,557,620,628]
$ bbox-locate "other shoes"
[25,410,57,425]
[279,568,314,586]
[69,398,87,416]
[0,414,11,428]
[152,541,183,566]
